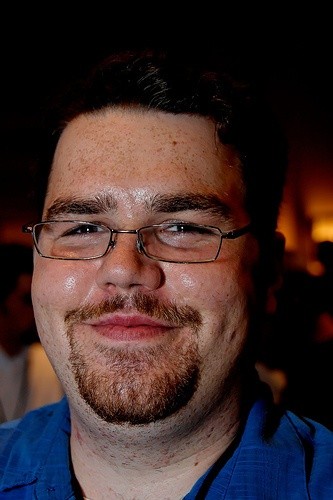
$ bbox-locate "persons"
[0,51,333,500]
[0,241,333,433]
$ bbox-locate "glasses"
[22,212,260,263]
